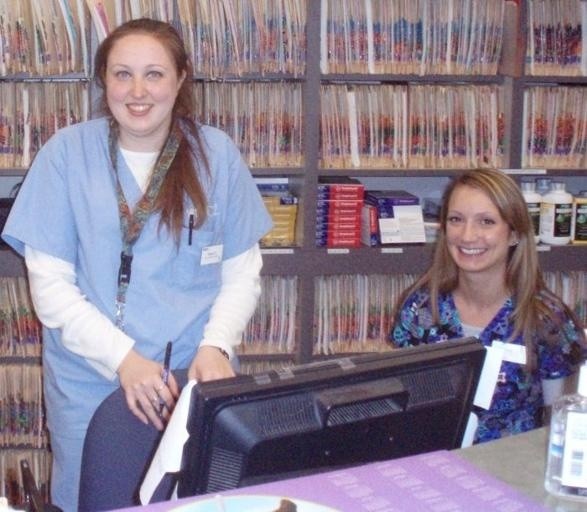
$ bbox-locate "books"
[234,273,297,376]
[540,270,586,321]
[1,276,52,511]
[314,274,426,353]
[2,1,303,169]
[256,182,298,249]
[317,1,586,169]
[316,177,446,250]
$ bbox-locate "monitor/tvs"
[178,337,487,497]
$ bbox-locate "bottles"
[572,190,586,244]
[543,365,586,503]
[541,182,573,244]
[521,181,541,244]
[535,178,552,195]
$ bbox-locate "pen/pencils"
[158,342,172,416]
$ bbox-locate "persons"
[1,17,276,510]
[387,169,586,447]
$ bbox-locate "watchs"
[219,348,228,359]
